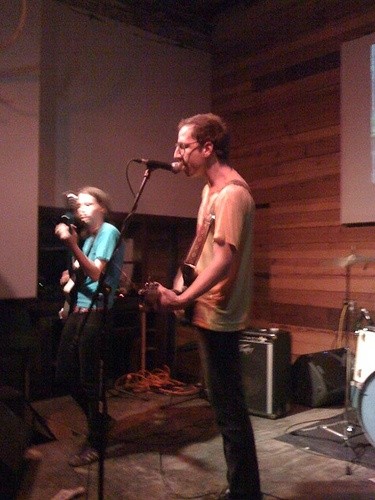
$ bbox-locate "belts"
[72,306,105,313]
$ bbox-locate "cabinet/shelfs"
[37,204,197,382]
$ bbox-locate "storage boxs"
[239,328,292,419]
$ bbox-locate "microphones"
[361,307,374,326]
[134,159,184,175]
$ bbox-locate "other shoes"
[87,413,116,441]
[212,489,231,500]
[68,446,99,466]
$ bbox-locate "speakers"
[293,348,356,408]
[0,385,57,500]
[236,329,292,418]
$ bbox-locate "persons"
[56,187,124,465]
[150,112,261,500]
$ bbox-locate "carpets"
[273,410,375,470]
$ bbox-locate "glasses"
[173,138,207,156]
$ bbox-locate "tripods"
[292,263,365,476]
